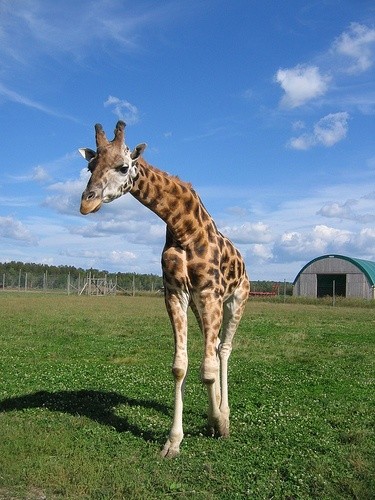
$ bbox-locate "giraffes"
[78,121,250,462]
